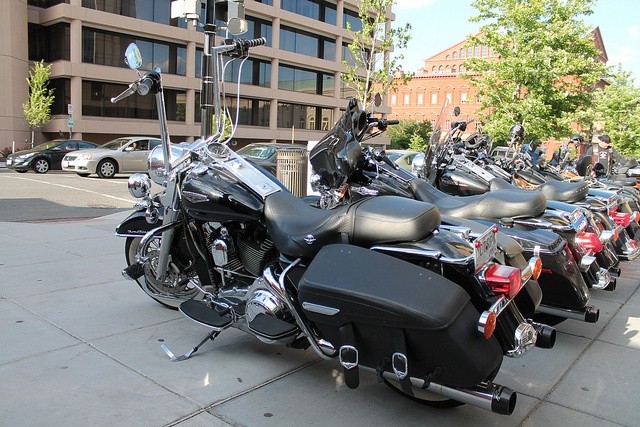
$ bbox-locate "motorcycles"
[110,18,558,414]
[296,100,601,349]
[406,95,622,292]
[485,116,639,263]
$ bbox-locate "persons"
[550,152,560,167]
[520,141,539,163]
[66,144,73,150]
[567,134,583,160]
[133,141,139,148]
[586,135,629,173]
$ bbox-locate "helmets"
[509,124,524,136]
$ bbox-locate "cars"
[384,148,417,169]
[233,143,309,172]
[6,139,101,173]
[61,136,171,178]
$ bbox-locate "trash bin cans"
[275,146,308,198]
